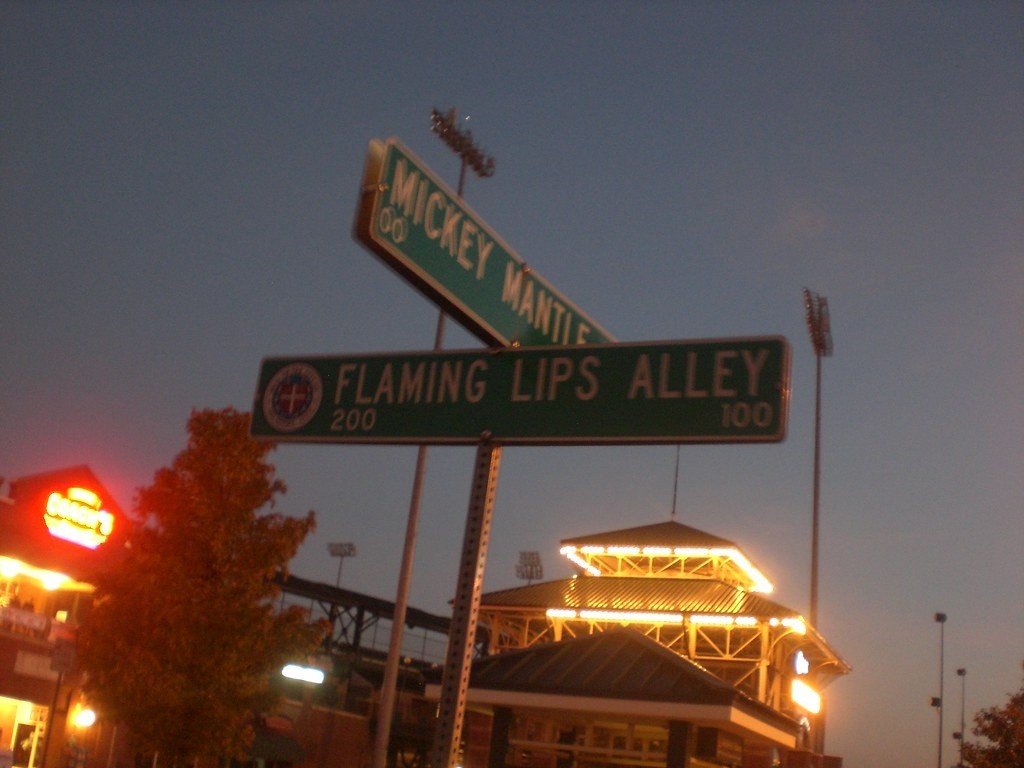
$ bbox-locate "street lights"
[932,612,948,768]
[954,667,965,768]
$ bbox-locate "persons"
[7,595,35,636]
[18,730,35,766]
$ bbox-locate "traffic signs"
[250,332,795,456]
[354,138,617,350]
